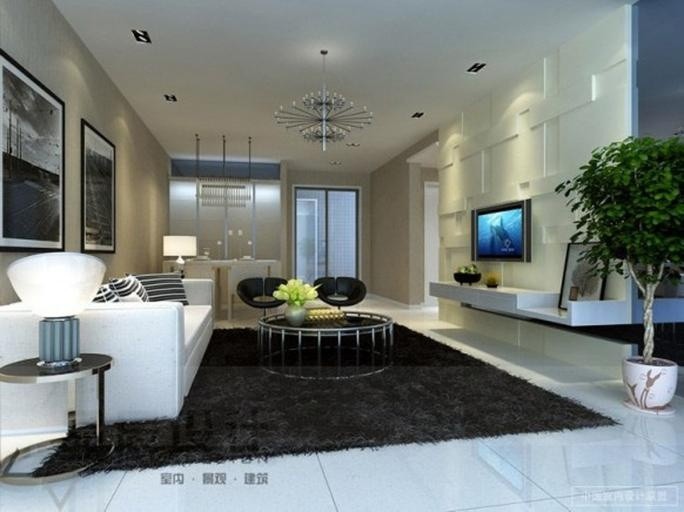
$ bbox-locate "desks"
[162,258,282,315]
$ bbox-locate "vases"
[284,303,308,327]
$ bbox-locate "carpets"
[37,322,622,479]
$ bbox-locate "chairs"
[170,260,215,281]
[313,276,367,312]
[236,277,287,321]
[227,262,271,321]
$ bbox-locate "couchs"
[1,279,216,425]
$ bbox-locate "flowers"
[273,278,322,305]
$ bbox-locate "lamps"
[274,49,379,154]
[163,236,197,274]
[6,252,106,369]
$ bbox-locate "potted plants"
[555,130,684,416]
[484,272,501,288]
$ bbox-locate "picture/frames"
[0,49,66,253]
[558,240,610,312]
[81,117,117,254]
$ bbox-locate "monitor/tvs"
[471,199,532,263]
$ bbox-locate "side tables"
[0,353,114,487]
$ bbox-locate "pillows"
[127,272,190,306]
[92,286,123,304]
[108,274,150,303]
